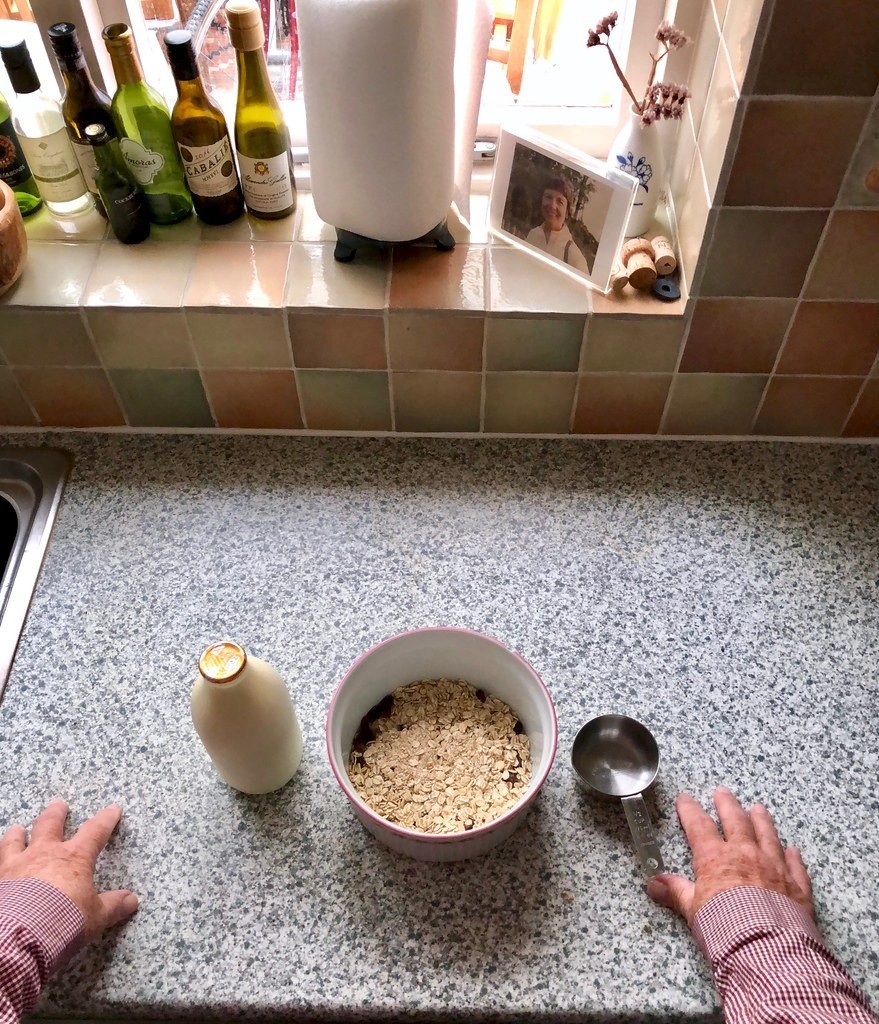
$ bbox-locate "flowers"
[585,9,693,127]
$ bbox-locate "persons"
[0,787,879,1024]
[526,179,590,276]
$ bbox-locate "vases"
[607,101,662,238]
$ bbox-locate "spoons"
[571,716,666,876]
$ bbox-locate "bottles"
[163,29,244,224]
[0,39,93,216]
[192,642,302,794]
[227,0,298,221]
[0,92,42,216]
[46,21,111,219]
[83,124,152,243]
[102,23,193,228]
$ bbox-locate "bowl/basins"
[0,179,28,298]
[326,627,559,861]
